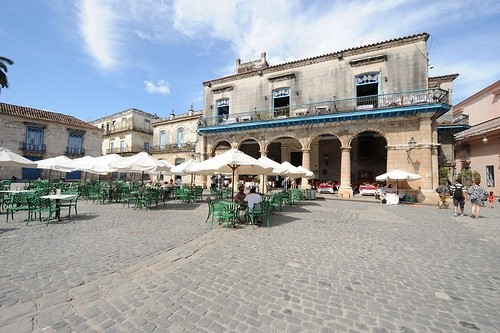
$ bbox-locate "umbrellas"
[0,146,175,186]
[171,146,315,202]
[375,169,421,194]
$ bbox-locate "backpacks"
[453,184,464,199]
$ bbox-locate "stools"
[405,193,413,202]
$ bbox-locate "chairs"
[0,178,383,232]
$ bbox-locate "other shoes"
[454,214,457,217]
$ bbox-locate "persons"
[450,179,467,216]
[211,175,216,188]
[234,186,247,209]
[468,179,487,219]
[436,181,450,207]
[489,191,495,208]
[160,176,182,199]
[145,180,153,189]
[243,188,262,224]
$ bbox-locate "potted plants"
[459,142,472,167]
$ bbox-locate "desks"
[34,194,79,219]
[220,200,246,225]
[384,192,399,206]
[358,184,376,196]
[0,190,38,213]
[316,183,334,194]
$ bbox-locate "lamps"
[482,134,489,144]
[406,136,418,152]
